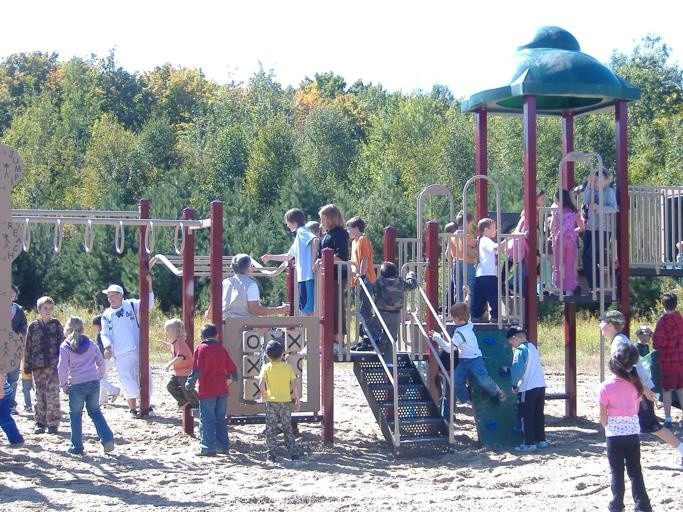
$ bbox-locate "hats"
[597,309,625,324]
[100,285,126,295]
[634,326,653,335]
[505,325,526,339]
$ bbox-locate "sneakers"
[216,449,228,454]
[266,456,278,462]
[350,342,363,352]
[497,390,505,402]
[129,409,138,418]
[515,443,537,452]
[291,455,301,462]
[103,440,114,452]
[34,425,45,434]
[356,344,374,351]
[148,407,155,416]
[66,446,84,454]
[110,388,120,404]
[194,450,215,456]
[47,426,58,434]
[535,441,548,447]
[10,407,18,416]
[99,397,107,404]
[21,406,31,412]
[663,421,673,432]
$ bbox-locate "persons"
[600,292,683,512]
[429,303,507,405]
[204,253,290,405]
[444,167,618,324]
[437,347,460,422]
[156,319,199,408]
[185,324,239,457]
[259,340,303,462]
[0,274,155,455]
[507,326,548,452]
[260,204,418,366]
